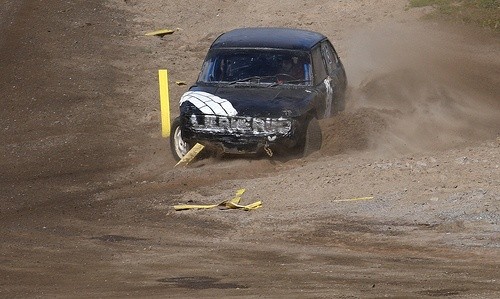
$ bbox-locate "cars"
[170,27,348,168]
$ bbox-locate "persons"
[277,56,304,83]
